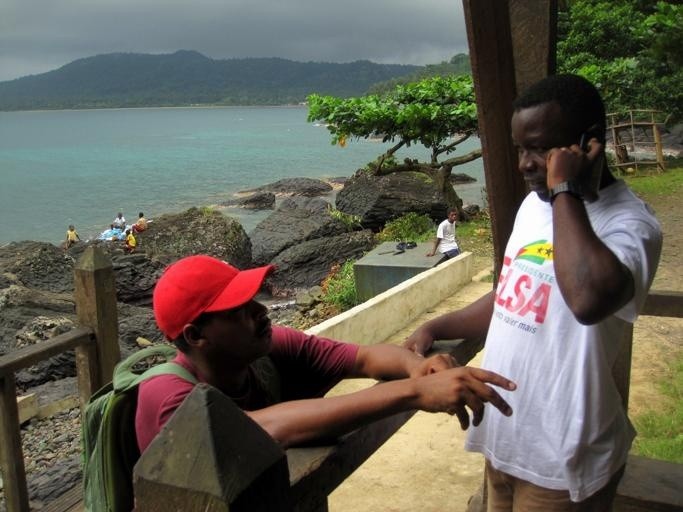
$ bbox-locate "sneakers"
[396,241,417,249]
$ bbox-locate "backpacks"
[79,343,201,511]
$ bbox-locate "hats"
[153,255,276,341]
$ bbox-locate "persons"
[134,255,516,456]
[65,224,81,250]
[426,207,462,258]
[401,74,663,512]
[111,212,148,253]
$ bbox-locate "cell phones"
[580,123,605,195]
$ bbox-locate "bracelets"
[549,181,584,206]
